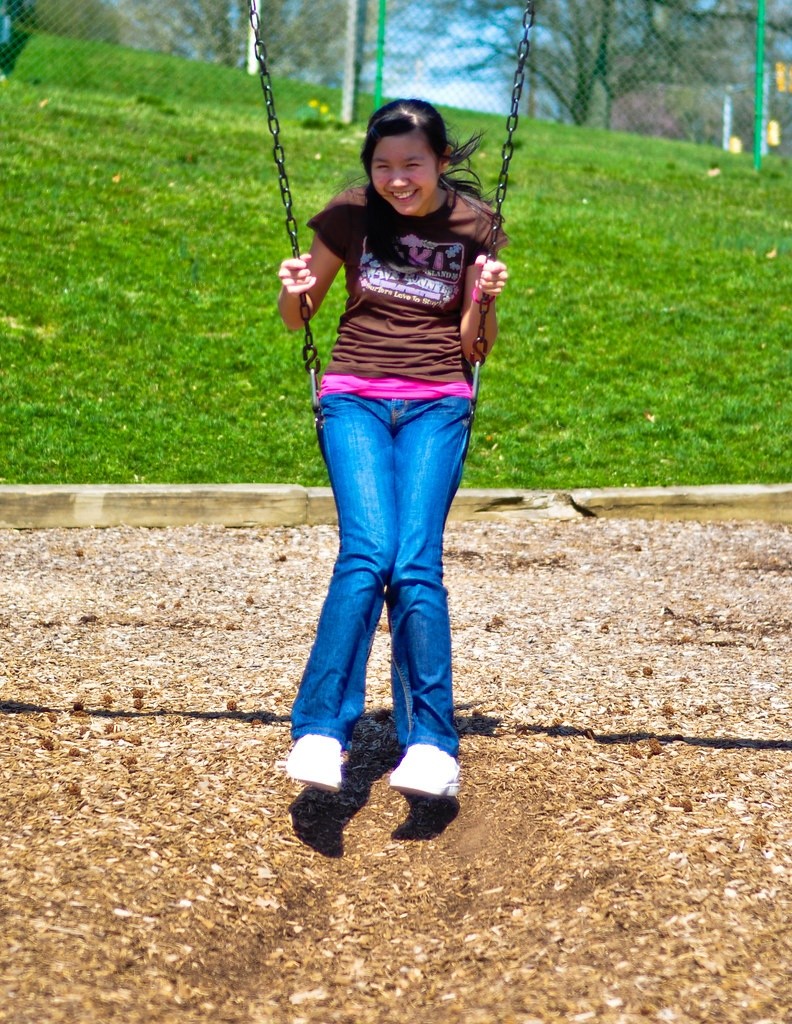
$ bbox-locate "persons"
[277,98,511,797]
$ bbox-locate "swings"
[247,0,539,457]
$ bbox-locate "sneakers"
[391,744,459,798]
[286,733,344,792]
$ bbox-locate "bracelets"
[472,289,495,305]
